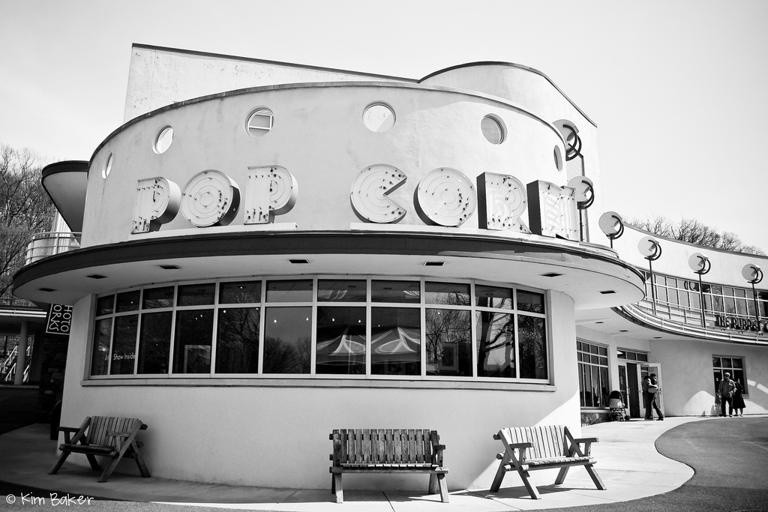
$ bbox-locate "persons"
[729,376,746,417]
[718,372,738,417]
[649,373,663,420]
[643,375,659,419]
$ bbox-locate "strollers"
[609,390,629,421]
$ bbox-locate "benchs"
[328,428,450,504]
[49,414,152,483]
[488,423,607,499]
